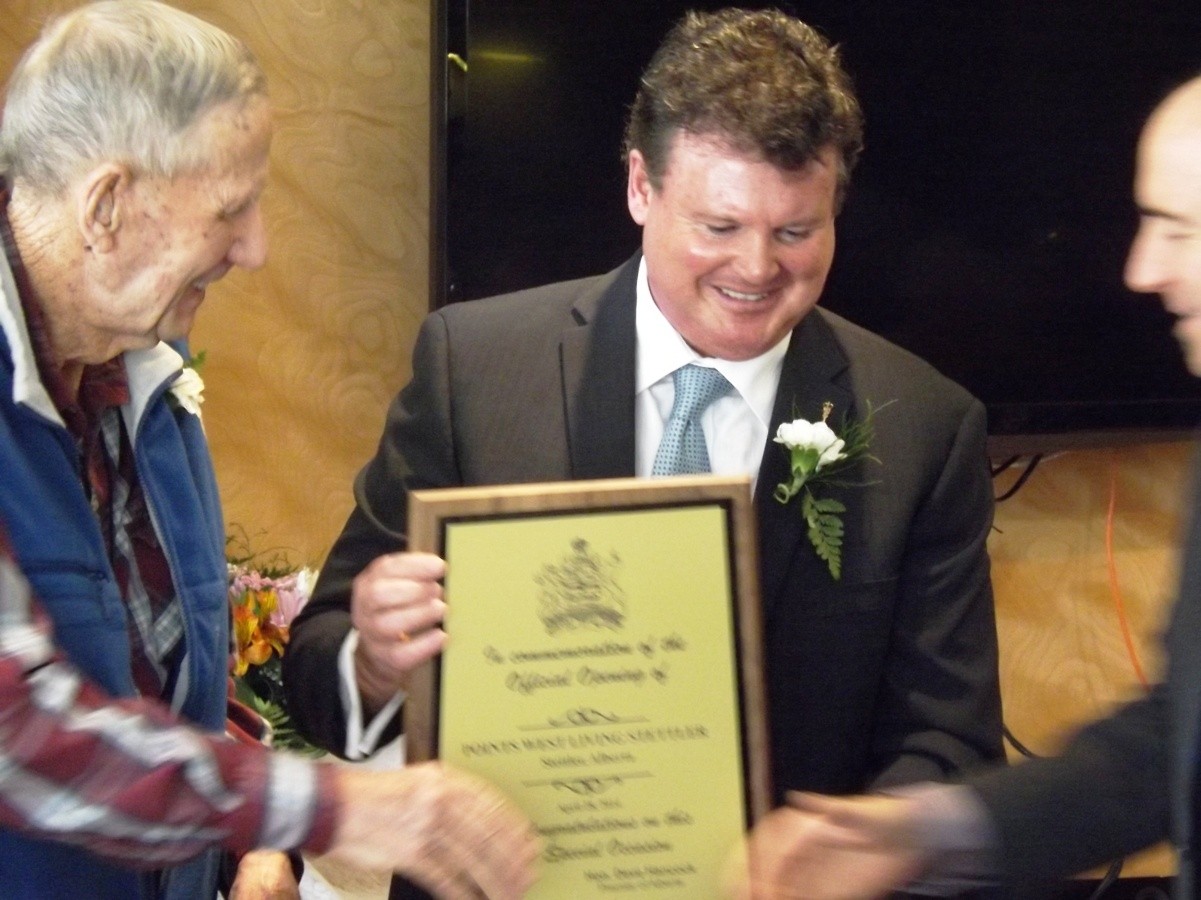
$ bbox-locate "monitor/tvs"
[427,1,1200,444]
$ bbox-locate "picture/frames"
[401,473,774,899]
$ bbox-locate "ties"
[651,364,735,478]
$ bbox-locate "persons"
[0,0,551,898]
[278,10,1012,900]
[743,72,1201,900]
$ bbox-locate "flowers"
[218,512,330,763]
[777,393,901,583]
